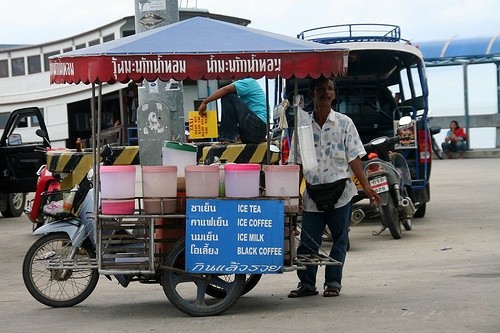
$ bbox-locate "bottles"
[76,138,82,152]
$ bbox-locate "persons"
[287,77,382,298]
[441,121,468,159]
[198,77,271,143]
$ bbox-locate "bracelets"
[203,99,208,105]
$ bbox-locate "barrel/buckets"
[141,165,178,214]
[264,165,300,213]
[100,164,135,215]
[185,166,220,199]
[224,163,262,197]
[161,140,198,190]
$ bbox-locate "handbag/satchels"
[304,178,351,211]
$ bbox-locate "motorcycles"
[353,115,423,240]
[427,114,444,160]
[23,15,351,316]
[282,24,433,218]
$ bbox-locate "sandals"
[288,285,319,298]
[323,286,340,297]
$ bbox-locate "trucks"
[0,106,53,218]
